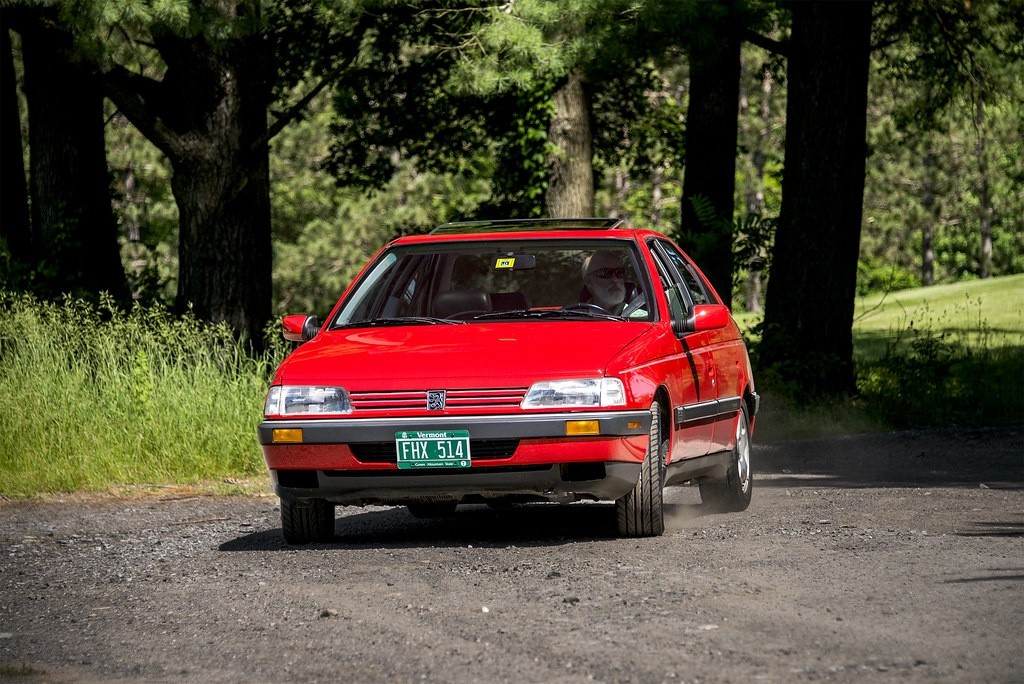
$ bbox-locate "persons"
[580,249,648,317]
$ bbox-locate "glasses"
[586,268,625,279]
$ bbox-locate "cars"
[256,215,759,544]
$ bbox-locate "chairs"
[579,283,638,309]
[488,292,528,313]
[431,289,492,321]
